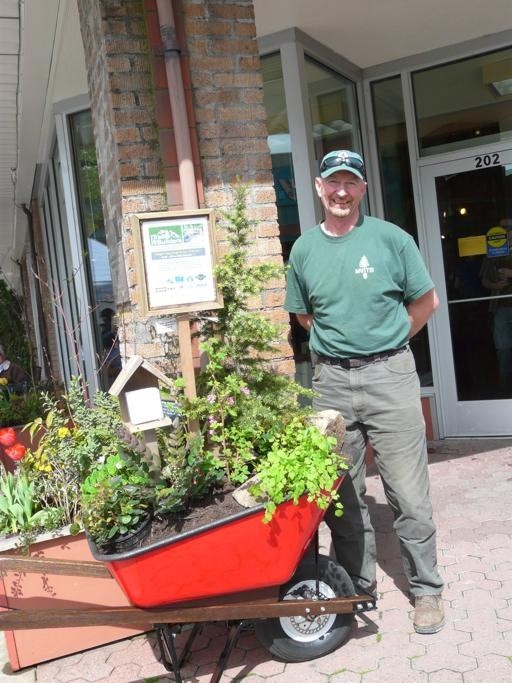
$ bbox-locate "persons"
[0,344,28,401]
[98,308,122,391]
[479,215,512,391]
[283,150,445,634]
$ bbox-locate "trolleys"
[0,453,379,683]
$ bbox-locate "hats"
[320,150,364,180]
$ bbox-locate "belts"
[320,344,406,367]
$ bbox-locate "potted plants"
[71,453,158,553]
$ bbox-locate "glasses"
[321,156,364,174]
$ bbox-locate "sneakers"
[414,595,446,634]
